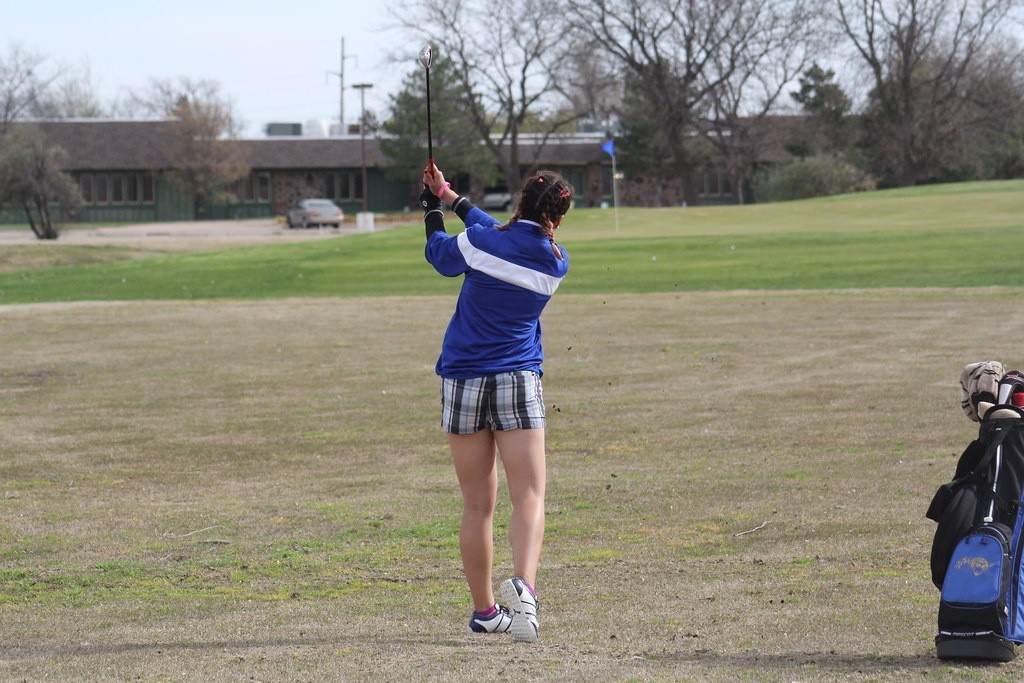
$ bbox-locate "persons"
[419,163,577,643]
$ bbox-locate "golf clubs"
[418,43,435,181]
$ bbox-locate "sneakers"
[467,605,539,634]
[499,576,540,643]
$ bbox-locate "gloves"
[419,186,444,217]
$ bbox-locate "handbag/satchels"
[1004,481,1024,644]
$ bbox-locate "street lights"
[351,82,377,232]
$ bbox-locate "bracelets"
[437,182,452,198]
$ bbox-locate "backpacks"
[926,421,1012,591]
[936,523,1019,662]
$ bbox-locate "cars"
[287,197,345,230]
[464,184,512,213]
